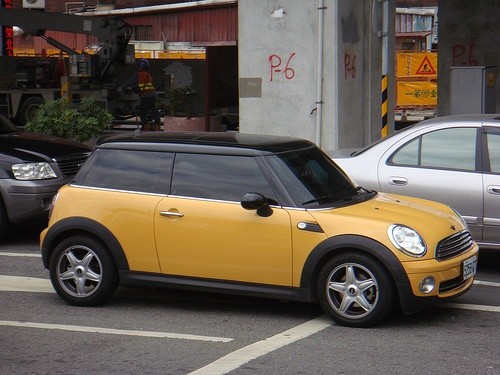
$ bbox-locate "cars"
[300,114,500,251]
[40,129,479,329]
[0,113,95,244]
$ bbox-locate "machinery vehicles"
[0,5,166,127]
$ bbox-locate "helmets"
[136,59,150,69]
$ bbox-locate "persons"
[117,58,161,132]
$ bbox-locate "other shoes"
[151,124,161,132]
[142,124,150,132]
